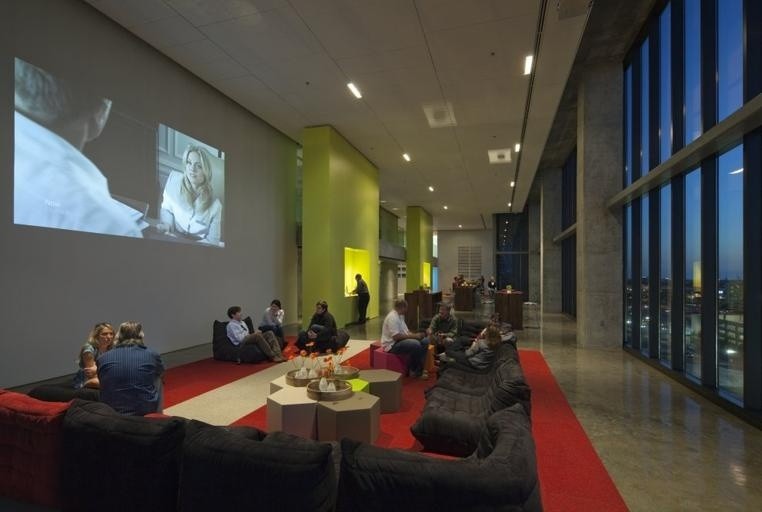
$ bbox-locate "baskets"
[286,365,360,402]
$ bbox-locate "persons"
[155,143,225,248]
[378,301,503,381]
[225,298,338,362]
[71,319,167,417]
[351,274,370,324]
[452,274,497,297]
[14,58,149,240]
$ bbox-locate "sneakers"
[410,370,429,380]
[269,353,287,362]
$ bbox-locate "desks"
[495,289,523,330]
[267,368,403,444]
[405,291,442,329]
[454,284,476,311]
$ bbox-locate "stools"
[369,340,435,375]
[525,302,541,329]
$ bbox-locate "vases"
[290,359,342,377]
[327,382,337,392]
[319,377,328,392]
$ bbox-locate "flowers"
[285,349,350,369]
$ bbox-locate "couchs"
[212,316,283,364]
[1,372,543,512]
[410,318,531,456]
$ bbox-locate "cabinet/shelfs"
[398,265,408,295]
